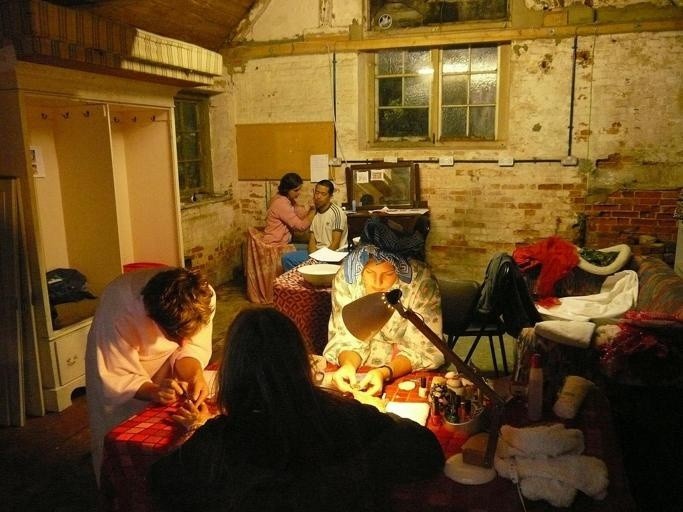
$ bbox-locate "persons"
[323,256,448,397]
[261,173,316,250]
[150,307,444,512]
[282,180,349,272]
[84,267,217,477]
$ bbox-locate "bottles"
[528,354,544,423]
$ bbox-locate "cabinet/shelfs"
[6,44,184,413]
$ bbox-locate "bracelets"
[380,365,392,382]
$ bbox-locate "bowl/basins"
[294,262,342,288]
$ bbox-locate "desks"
[271,247,357,354]
[102,363,635,512]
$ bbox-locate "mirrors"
[346,162,422,209]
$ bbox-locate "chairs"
[438,253,513,376]
[519,254,683,401]
